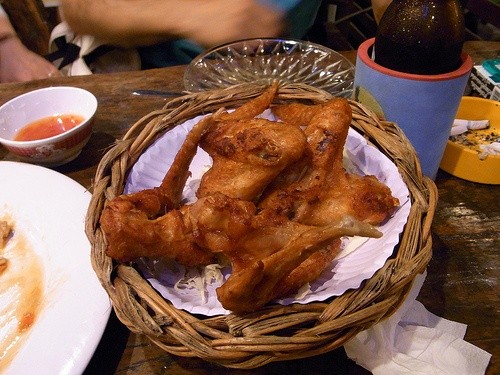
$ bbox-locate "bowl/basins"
[0,85,99,166]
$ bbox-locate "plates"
[182,36,356,100]
[0,159,111,375]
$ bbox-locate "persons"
[0,0,500,85]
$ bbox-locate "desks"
[0,39,500,375]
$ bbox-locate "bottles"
[370,0,463,76]
